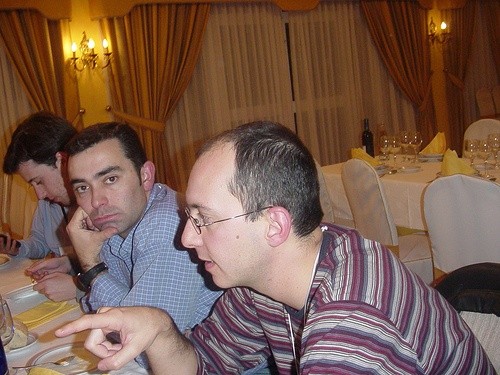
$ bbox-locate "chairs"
[313,119,500,375]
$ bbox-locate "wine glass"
[464,134,500,181]
[381,130,422,170]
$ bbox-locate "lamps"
[428,15,449,45]
[69,31,112,72]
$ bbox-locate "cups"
[0,336,10,375]
[0,294,14,345]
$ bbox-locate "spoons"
[11,356,75,370]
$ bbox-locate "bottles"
[362,118,375,158]
[379,120,387,154]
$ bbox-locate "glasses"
[184,206,273,235]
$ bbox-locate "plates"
[474,164,494,170]
[417,154,443,161]
[25,341,111,375]
[0,254,12,267]
[400,169,422,172]
[6,332,39,353]
[6,287,48,316]
[376,165,387,175]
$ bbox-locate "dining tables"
[319,157,500,232]
[0,251,153,375]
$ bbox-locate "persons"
[0,111,78,259]
[25,255,86,303]
[53,122,497,375]
[64,122,272,375]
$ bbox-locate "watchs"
[76,262,108,291]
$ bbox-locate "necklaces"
[288,226,328,375]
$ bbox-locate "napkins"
[420,132,447,154]
[351,148,382,167]
[14,299,77,330]
[440,148,475,176]
